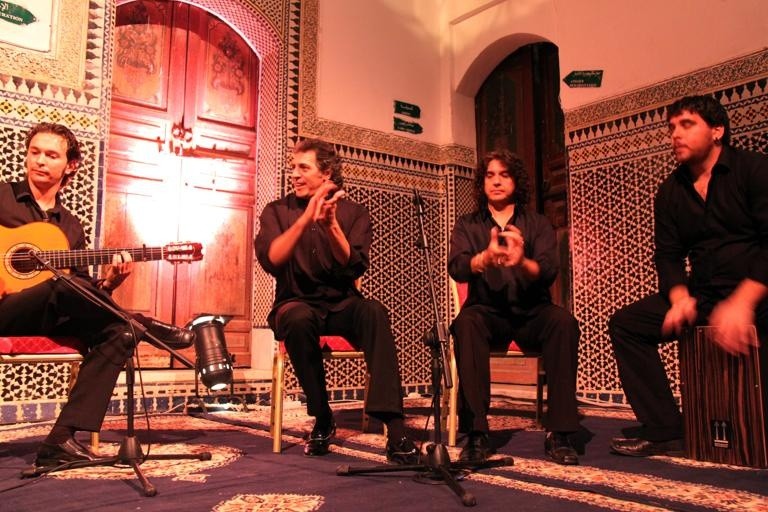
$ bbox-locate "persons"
[255,138,424,462]
[609,95,768,458]
[0,121,198,471]
[447,146,583,465]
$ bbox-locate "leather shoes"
[305,424,335,456]
[140,316,197,348]
[545,433,580,465]
[610,438,687,457]
[458,434,491,462]
[386,435,419,462]
[35,439,113,467]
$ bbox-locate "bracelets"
[474,250,489,274]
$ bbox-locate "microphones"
[28,249,37,258]
[414,188,423,214]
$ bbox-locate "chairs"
[0,329,110,449]
[444,264,548,449]
[268,274,389,455]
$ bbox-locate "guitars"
[0,222,203,297]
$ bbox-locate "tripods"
[335,332,514,506]
[21,356,212,497]
